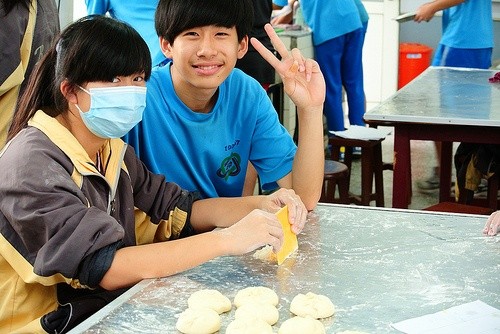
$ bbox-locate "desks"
[64,203,500,334]
[363,63,500,209]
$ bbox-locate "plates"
[395,9,417,23]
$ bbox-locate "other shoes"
[351,146,362,156]
[326,147,347,163]
[414,174,443,193]
[450,175,489,197]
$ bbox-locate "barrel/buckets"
[399,42,433,89]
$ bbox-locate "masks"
[69,84,148,139]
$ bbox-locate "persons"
[121,0,327,212]
[0,0,63,154]
[270,0,370,162]
[83,0,174,68]
[235,0,276,197]
[0,12,308,334]
[414,0,494,191]
[483,210,500,236]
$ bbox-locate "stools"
[327,130,385,209]
[319,161,349,205]
[425,202,495,216]
